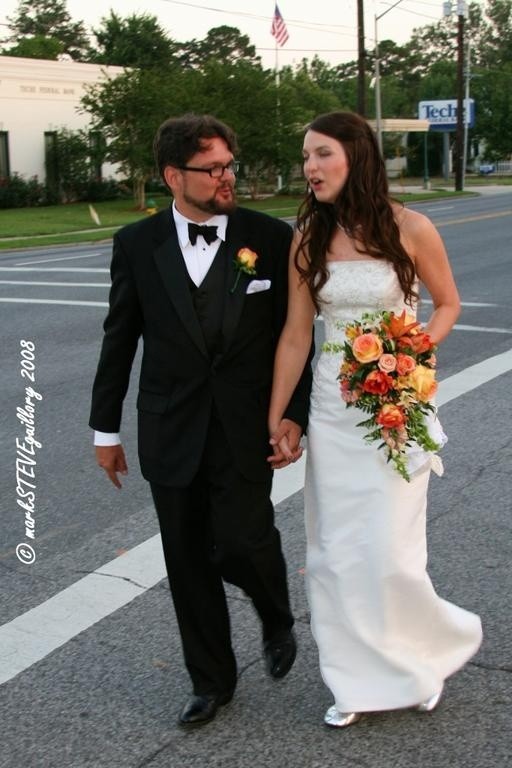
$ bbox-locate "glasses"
[184,159,242,179]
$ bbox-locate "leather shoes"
[177,692,228,728]
[264,632,297,678]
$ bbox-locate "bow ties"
[187,223,218,245]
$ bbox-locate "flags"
[270,3,289,48]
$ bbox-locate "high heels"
[323,704,360,727]
[419,683,445,713]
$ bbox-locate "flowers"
[320,309,450,485]
[228,245,258,295]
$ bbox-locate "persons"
[266,111,483,728]
[87,113,315,731]
